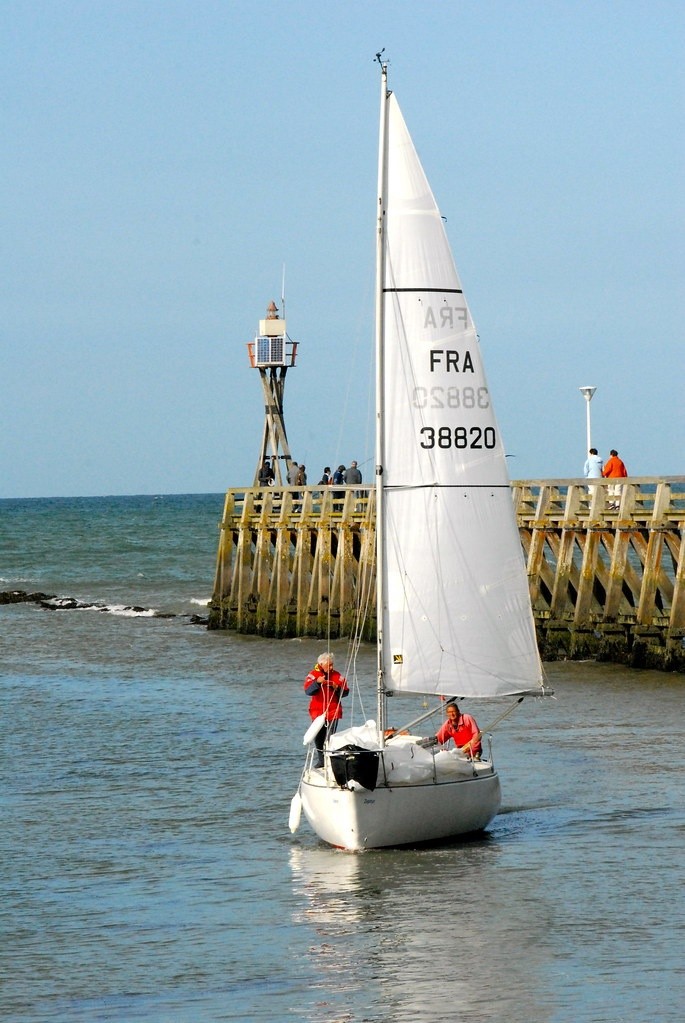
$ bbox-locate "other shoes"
[616,506,619,510]
[607,505,615,509]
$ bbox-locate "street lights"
[579,384,596,461]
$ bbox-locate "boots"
[314,751,324,768]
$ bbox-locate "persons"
[601,449,627,511]
[584,449,605,510]
[322,462,362,512]
[256,462,275,513]
[435,703,482,758]
[286,462,307,513]
[304,652,350,768]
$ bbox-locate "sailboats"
[284,40,557,855]
